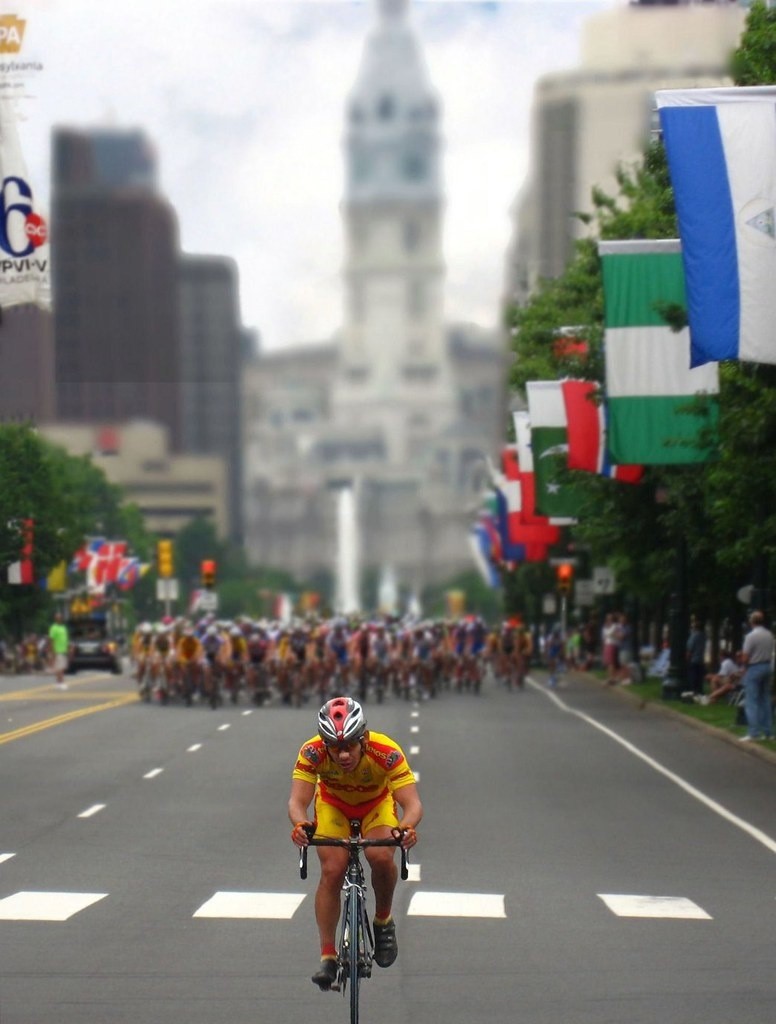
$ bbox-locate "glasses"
[324,738,360,753]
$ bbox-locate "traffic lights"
[202,561,215,590]
[550,557,579,596]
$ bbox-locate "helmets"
[318,697,367,744]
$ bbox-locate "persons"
[287,697,424,990]
[1,609,750,723]
[737,610,776,742]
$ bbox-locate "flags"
[470,324,647,587]
[596,237,721,464]
[5,519,154,604]
[1,6,53,317]
[655,84,776,369]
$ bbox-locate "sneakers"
[373,917,398,968]
[312,958,337,983]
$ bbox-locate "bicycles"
[294,822,412,1024]
[130,616,569,709]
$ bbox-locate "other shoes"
[739,735,765,742]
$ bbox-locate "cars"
[63,618,124,676]
